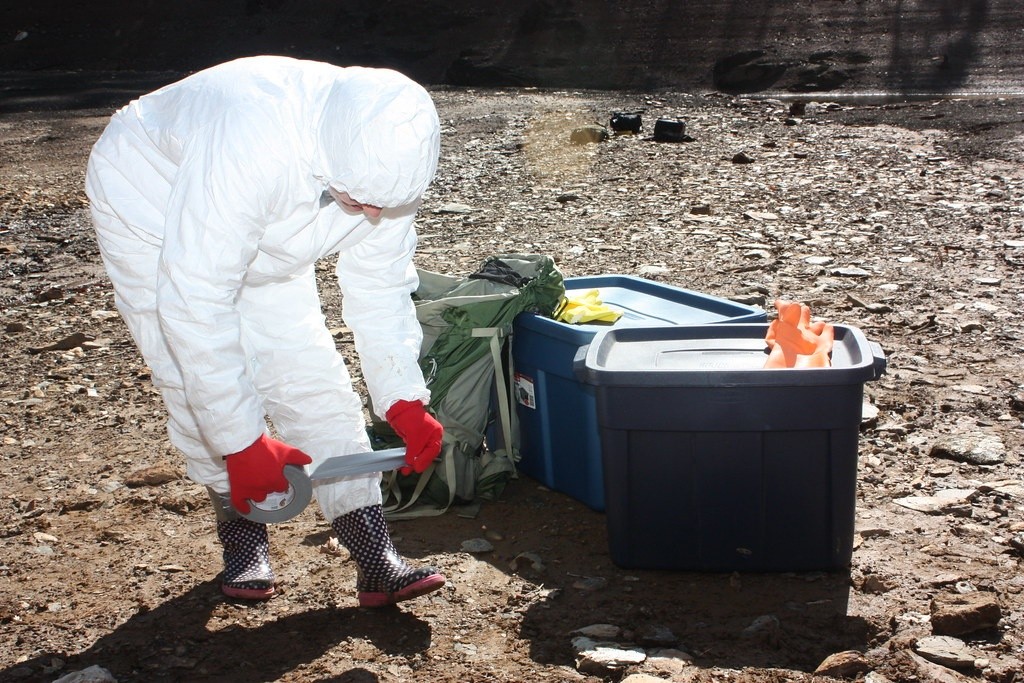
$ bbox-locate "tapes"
[228,458,314,526]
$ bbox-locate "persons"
[83,56,448,607]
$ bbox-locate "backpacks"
[362,253,570,523]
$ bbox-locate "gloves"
[762,299,834,371]
[386,398,444,476]
[554,289,623,323]
[225,433,312,516]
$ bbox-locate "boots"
[215,493,274,600]
[330,504,446,607]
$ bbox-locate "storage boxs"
[572,323,887,573]
[512,273,768,511]
